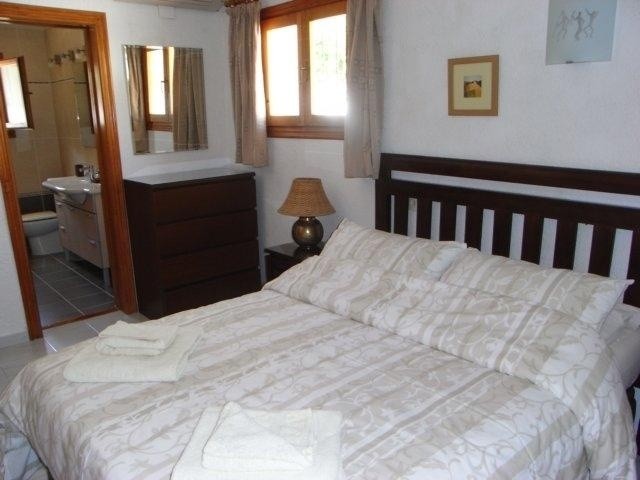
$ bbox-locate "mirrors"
[71,48,95,148]
[124,44,208,151]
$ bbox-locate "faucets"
[80,163,94,179]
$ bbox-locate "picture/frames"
[447,56,498,115]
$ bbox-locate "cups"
[75,165,84,177]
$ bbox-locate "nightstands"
[264,241,324,286]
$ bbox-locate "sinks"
[43,178,89,205]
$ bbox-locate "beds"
[1,152,640,480]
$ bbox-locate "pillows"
[404,247,632,342]
[322,216,466,345]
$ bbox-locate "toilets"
[21,209,66,255]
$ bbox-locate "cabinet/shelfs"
[51,189,110,286]
[121,165,262,319]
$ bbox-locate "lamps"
[273,175,334,247]
